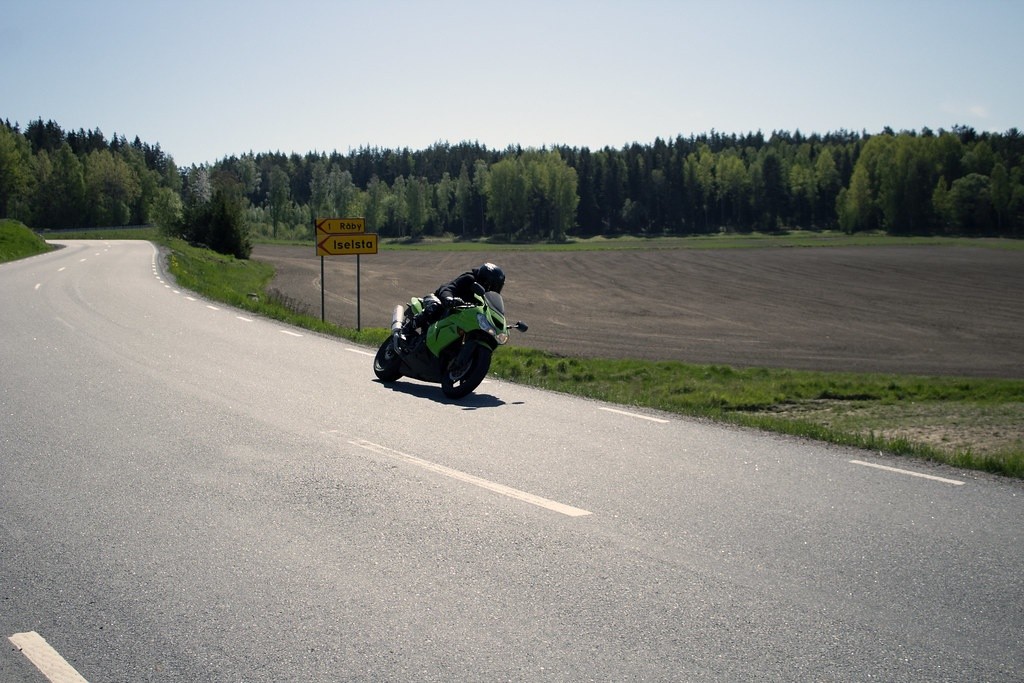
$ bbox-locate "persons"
[397,262,506,347]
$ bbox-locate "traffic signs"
[317,234,377,257]
[314,217,365,234]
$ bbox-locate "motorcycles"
[374,279,529,400]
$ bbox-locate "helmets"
[478,263,505,293]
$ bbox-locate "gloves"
[448,297,465,308]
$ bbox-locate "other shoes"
[401,319,416,346]
